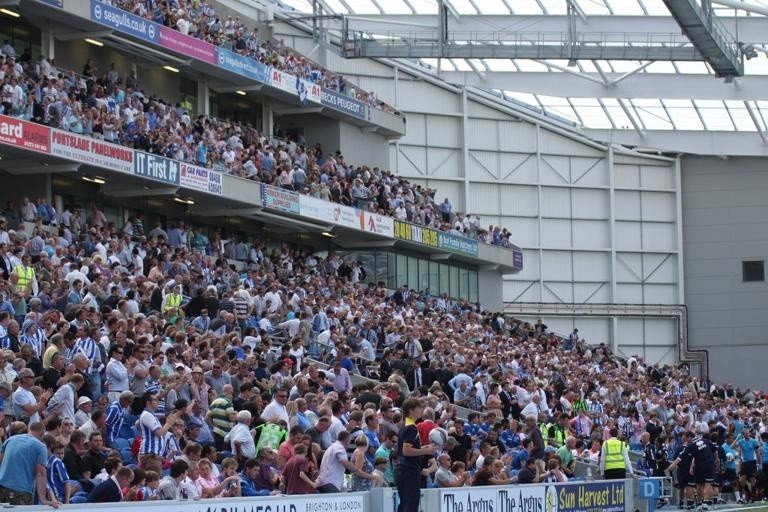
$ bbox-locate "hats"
[36,310,60,326]
[192,367,203,374]
[187,422,202,431]
[23,320,34,332]
[544,446,558,453]
[78,396,91,405]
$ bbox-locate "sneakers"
[717,495,749,504]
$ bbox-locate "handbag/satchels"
[256,423,286,452]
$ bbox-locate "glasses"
[213,365,220,369]
